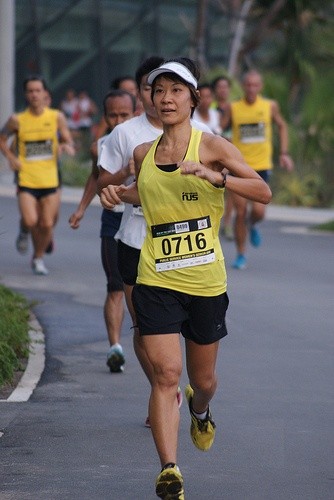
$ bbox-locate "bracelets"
[211,172,227,188]
[280,151,288,156]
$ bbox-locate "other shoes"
[233,254,247,269]
[145,387,183,427]
[223,224,239,240]
[249,224,261,247]
[30,259,49,274]
[16,228,29,255]
[105,343,125,372]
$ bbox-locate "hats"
[146,61,199,89]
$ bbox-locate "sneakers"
[154,462,185,500]
[185,384,217,452]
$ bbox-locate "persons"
[0,58,289,426]
[99,58,272,500]
